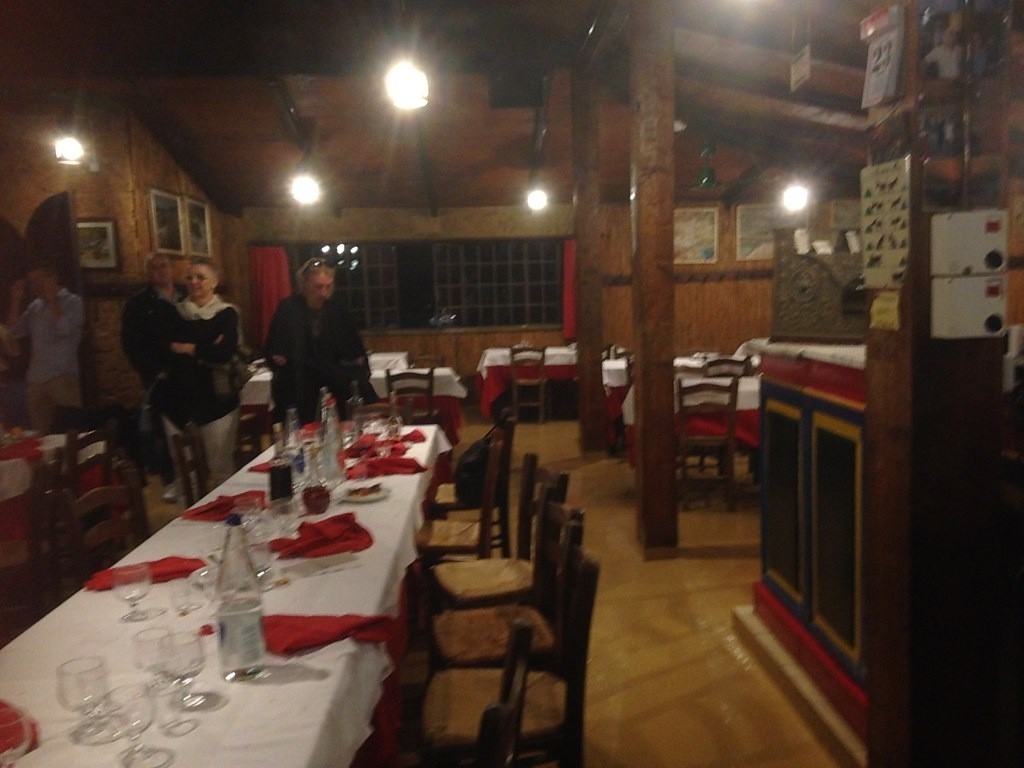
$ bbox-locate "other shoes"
[164,482,181,502]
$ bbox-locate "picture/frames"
[146,186,213,261]
[76,217,122,273]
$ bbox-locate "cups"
[235,496,263,521]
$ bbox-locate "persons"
[264,258,370,427]
[8,259,83,430]
[121,250,185,501]
[163,257,241,509]
[919,25,971,79]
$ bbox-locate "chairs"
[26,342,761,768]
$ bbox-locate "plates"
[333,479,390,502]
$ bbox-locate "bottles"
[303,458,330,513]
[268,422,293,502]
[387,391,403,437]
[213,514,270,682]
[319,386,345,481]
[346,380,364,440]
[286,407,302,449]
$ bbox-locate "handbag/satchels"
[456,418,505,505]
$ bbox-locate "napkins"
[84,557,205,591]
[250,513,373,560]
[0,439,42,463]
[181,490,266,522]
[343,430,425,458]
[346,458,429,480]
[248,462,271,472]
[201,614,400,658]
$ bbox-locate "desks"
[239,351,408,434]
[327,366,467,444]
[0,423,452,768]
[475,346,578,419]
[621,377,761,469]
[0,434,119,544]
[602,356,759,450]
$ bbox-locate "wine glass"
[111,563,150,622]
[55,628,205,767]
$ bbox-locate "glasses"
[304,260,335,273]
